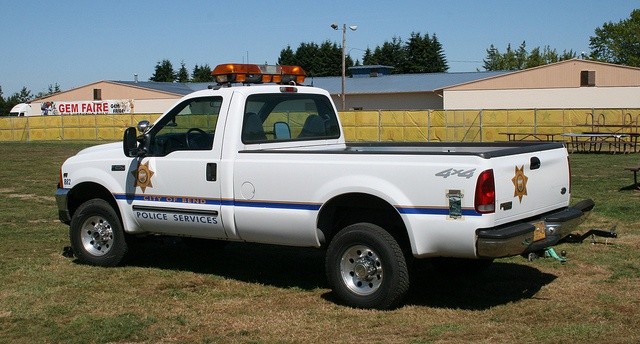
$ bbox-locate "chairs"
[298,114,326,138]
[242,112,266,144]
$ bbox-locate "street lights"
[331,22,358,110]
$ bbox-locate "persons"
[41,101,54,116]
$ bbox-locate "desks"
[560,133,631,155]
[498,132,561,142]
[582,135,640,155]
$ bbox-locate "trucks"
[55,63,596,309]
[8,98,134,116]
